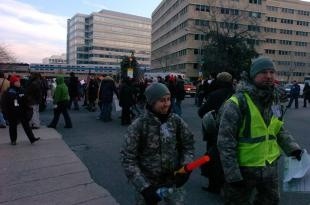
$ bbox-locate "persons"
[285,80,300,109]
[0,71,234,205]
[303,82,310,107]
[216,56,304,205]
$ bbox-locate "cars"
[183,78,197,97]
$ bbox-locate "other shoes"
[12,141,16,144]
[64,126,72,128]
[0,125,6,128]
[73,108,79,110]
[48,125,56,128]
[31,138,39,143]
[30,126,40,129]
[202,186,219,194]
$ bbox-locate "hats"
[250,58,276,79]
[10,76,19,84]
[145,83,171,106]
[217,72,232,82]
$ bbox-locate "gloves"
[230,180,249,192]
[173,173,190,187]
[291,149,303,160]
[141,185,162,205]
